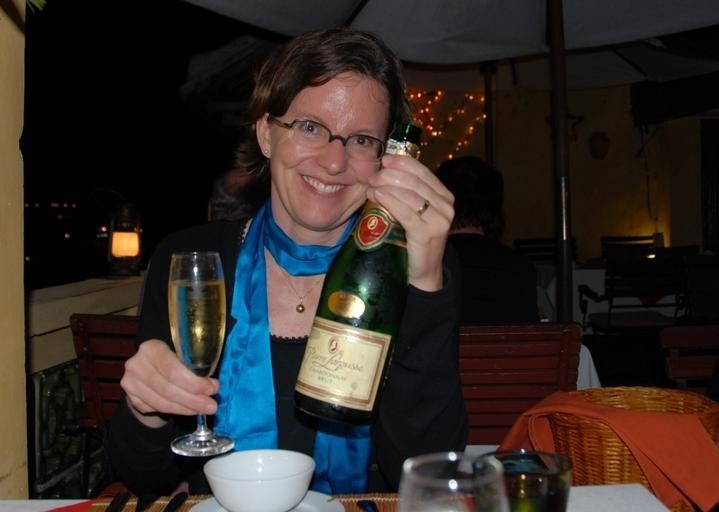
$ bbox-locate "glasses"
[265,115,396,163]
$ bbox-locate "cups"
[472,449,573,512]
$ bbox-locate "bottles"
[291,121,424,425]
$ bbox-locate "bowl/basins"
[203,449,317,512]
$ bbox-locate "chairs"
[515,236,564,263]
[575,235,690,331]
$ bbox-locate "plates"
[187,490,346,512]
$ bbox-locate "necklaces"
[280,270,322,313]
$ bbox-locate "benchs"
[662,329,719,404]
[70,314,582,496]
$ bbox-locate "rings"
[417,200,431,215]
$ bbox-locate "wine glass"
[169,251,235,459]
[395,451,512,512]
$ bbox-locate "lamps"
[107,217,141,277]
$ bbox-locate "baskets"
[546,387,717,496]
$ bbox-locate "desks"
[84,484,666,511]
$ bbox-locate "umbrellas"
[187,0,719,323]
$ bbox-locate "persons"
[105,27,468,495]
[434,157,539,322]
